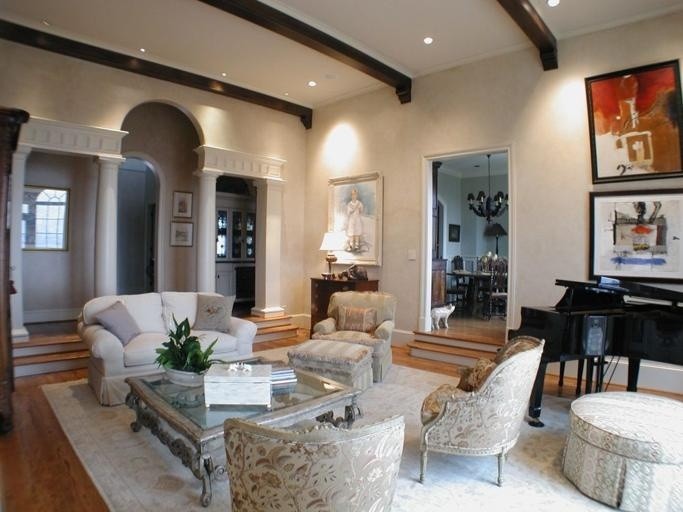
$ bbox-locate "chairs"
[310,292,397,383]
[418,336,546,485]
[222,417,405,512]
[446,264,508,321]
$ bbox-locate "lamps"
[319,233,344,279]
[484,225,508,254]
[466,154,508,223]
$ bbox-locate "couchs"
[75,291,256,407]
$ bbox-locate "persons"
[346,189,364,251]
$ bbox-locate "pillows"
[466,355,497,392]
[191,294,236,333]
[335,305,377,333]
[93,301,141,346]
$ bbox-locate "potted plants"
[152,314,226,387]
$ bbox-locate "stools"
[561,392,683,512]
[288,340,374,396]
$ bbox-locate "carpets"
[42,345,615,512]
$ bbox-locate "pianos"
[508,276,683,427]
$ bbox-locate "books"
[270,385,296,396]
[257,360,297,384]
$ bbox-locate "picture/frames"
[584,58,683,183]
[449,224,460,242]
[329,174,383,267]
[589,192,683,284]
[170,222,194,246]
[172,191,193,218]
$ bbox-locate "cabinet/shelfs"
[310,278,379,338]
[215,192,256,303]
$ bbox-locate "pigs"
[431,303,456,331]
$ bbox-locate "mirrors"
[21,184,72,254]
